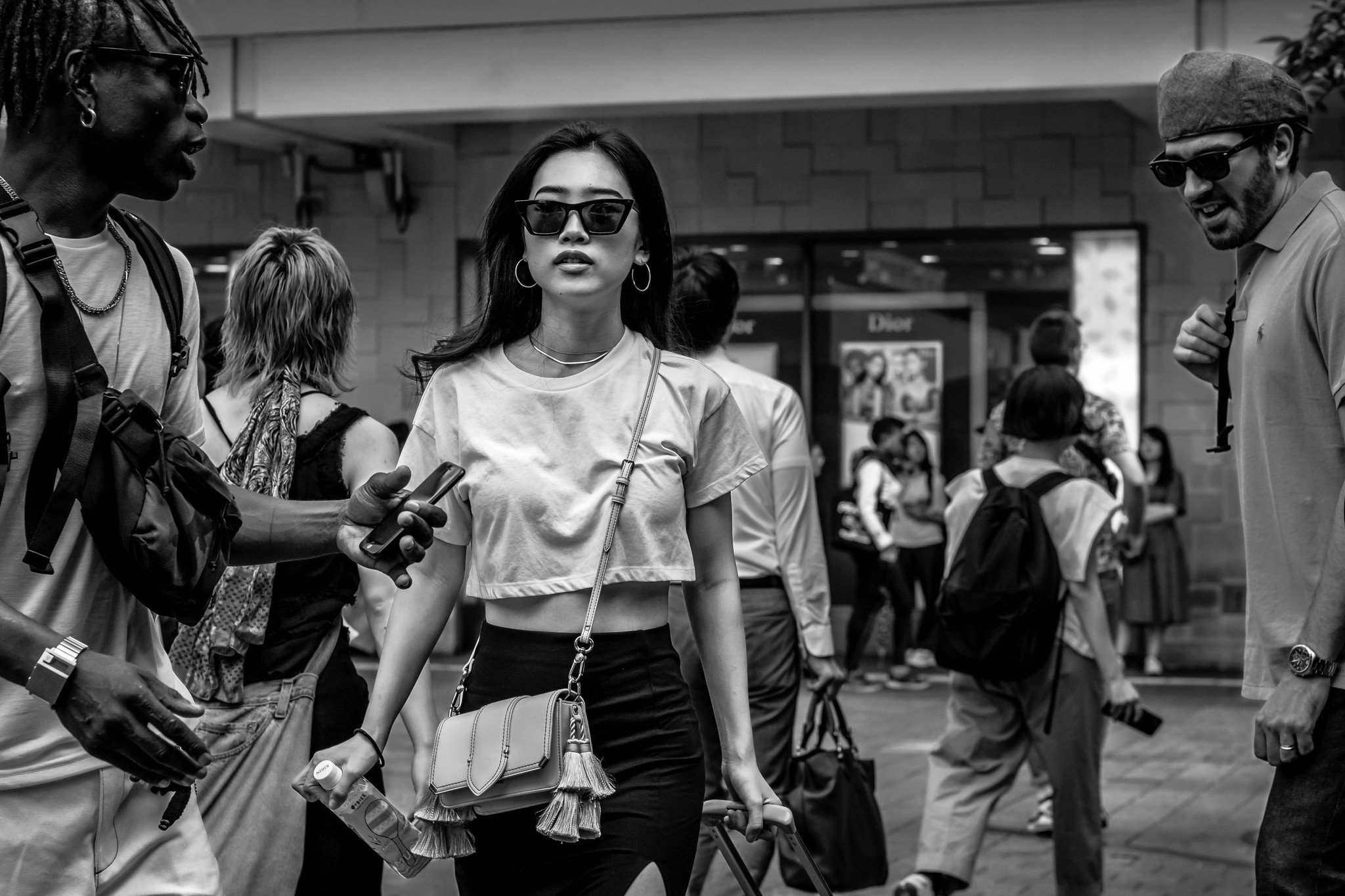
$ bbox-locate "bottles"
[312,760,432,878]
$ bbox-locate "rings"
[1279,744,1297,750]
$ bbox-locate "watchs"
[25,636,89,710]
[1288,644,1339,678]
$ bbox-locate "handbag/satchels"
[407,686,617,860]
[776,690,890,892]
[192,671,320,896]
[836,451,892,544]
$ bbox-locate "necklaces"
[527,332,613,366]
[0,176,131,388]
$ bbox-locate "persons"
[1150,50,1345,896]
[893,365,1140,896]
[849,416,948,693]
[0,0,447,896]
[980,309,1192,837]
[157,228,438,896]
[292,120,782,896]
[844,348,940,427]
[672,242,847,896]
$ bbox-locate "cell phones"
[1100,702,1164,737]
[358,461,466,559]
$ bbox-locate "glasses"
[1147,139,1267,187]
[511,199,638,236]
[66,48,199,103]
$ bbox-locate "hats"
[1158,51,1312,140]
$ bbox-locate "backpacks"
[931,463,1071,686]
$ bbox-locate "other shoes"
[839,659,933,690]
[893,873,935,896]
[1026,796,1054,831]
[1145,658,1163,674]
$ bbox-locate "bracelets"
[353,728,385,769]
